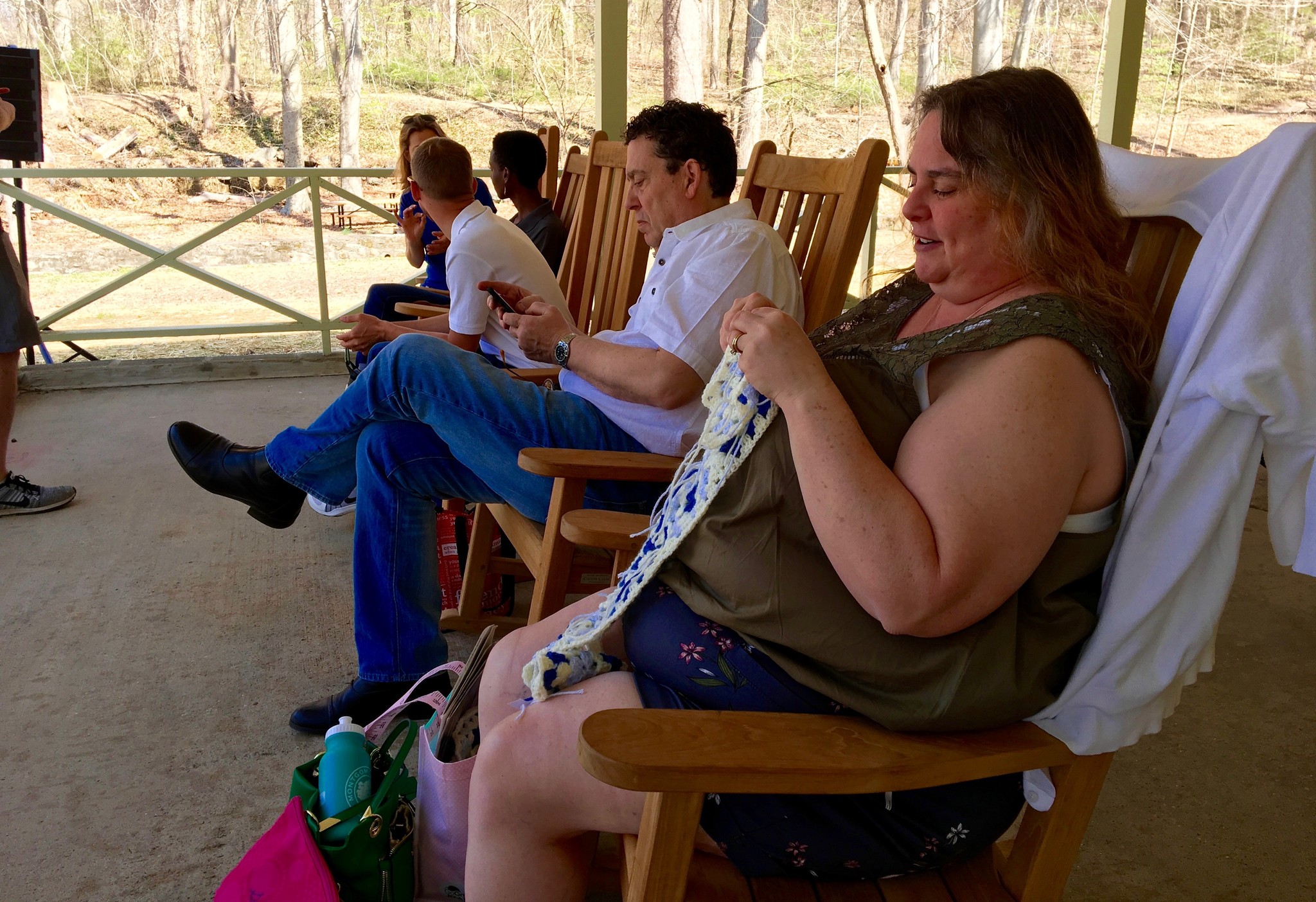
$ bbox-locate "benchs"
[336,211,400,231]
[320,207,400,228]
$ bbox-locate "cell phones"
[486,286,516,314]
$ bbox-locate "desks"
[377,188,405,209]
[331,197,401,230]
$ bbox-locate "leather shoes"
[167,420,308,529]
[289,669,453,735]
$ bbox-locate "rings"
[730,331,746,356]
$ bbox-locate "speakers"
[0,46,44,162]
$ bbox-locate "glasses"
[407,176,423,193]
[400,114,438,129]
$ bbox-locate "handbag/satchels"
[437,507,509,621]
[361,660,476,902]
[288,717,418,902]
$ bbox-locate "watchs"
[551,333,579,366]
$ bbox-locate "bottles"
[319,716,371,839]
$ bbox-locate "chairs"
[392,115,1316,902]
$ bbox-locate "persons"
[166,98,807,739]
[463,66,1150,902]
[0,44,76,518]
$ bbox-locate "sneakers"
[0,470,76,516]
[308,485,357,518]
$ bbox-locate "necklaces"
[913,285,1007,335]
